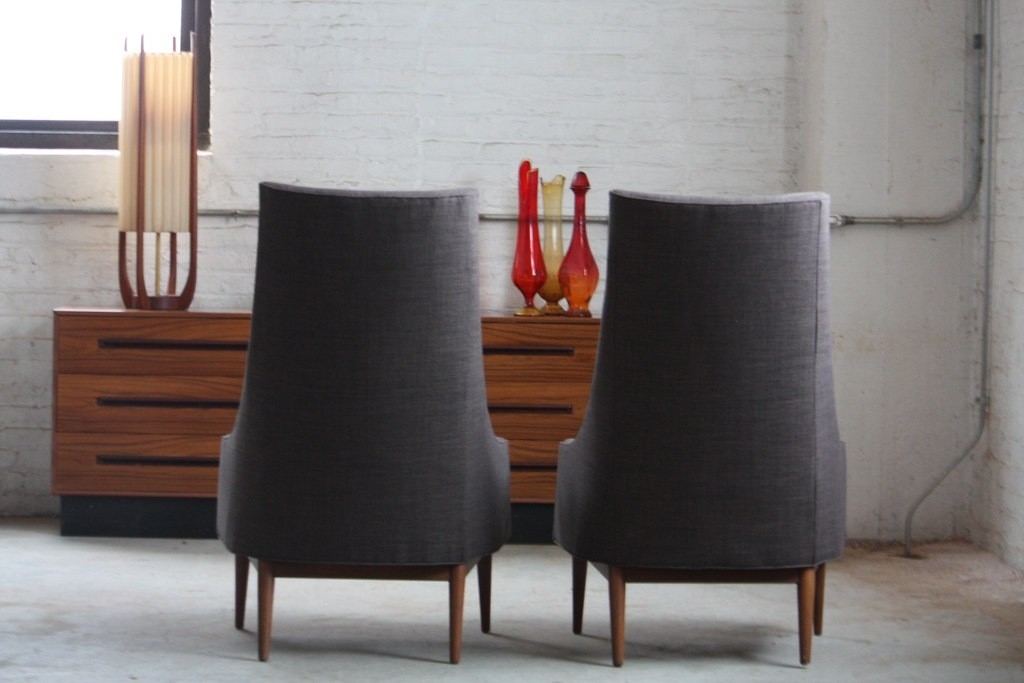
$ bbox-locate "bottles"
[558,171,599,317]
[510,159,548,317]
[538,175,567,316]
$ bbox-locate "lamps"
[115,31,198,314]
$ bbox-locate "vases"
[511,161,601,319]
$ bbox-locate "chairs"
[215,181,510,666]
[552,188,848,671]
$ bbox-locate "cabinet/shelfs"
[41,303,601,546]
